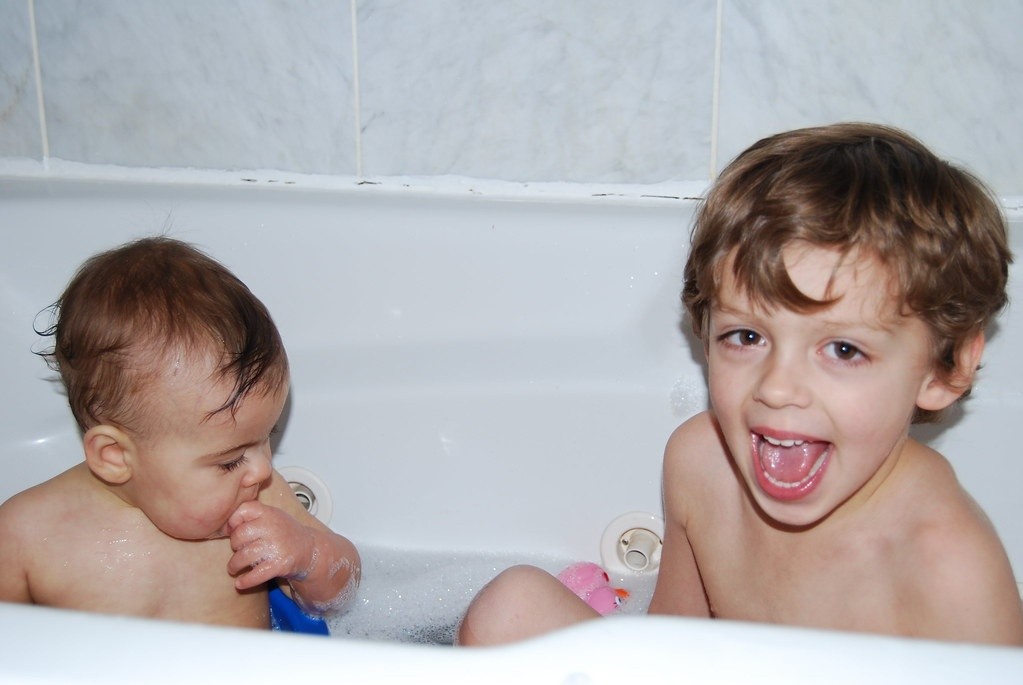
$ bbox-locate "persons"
[0,236,360,630]
[461,122,1023,650]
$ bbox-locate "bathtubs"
[1,156,1023,685]
